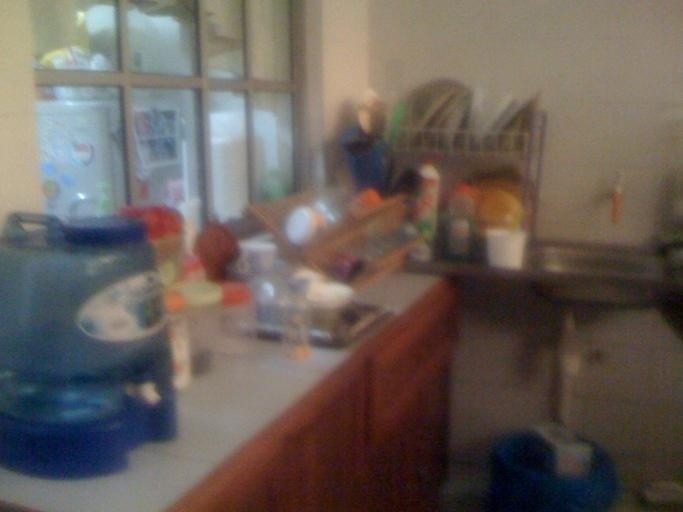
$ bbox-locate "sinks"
[531,245,667,306]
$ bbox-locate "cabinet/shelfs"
[0,343,373,512]
[387,99,548,168]
[365,285,457,510]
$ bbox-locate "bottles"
[410,157,476,262]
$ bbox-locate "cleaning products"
[409,153,444,263]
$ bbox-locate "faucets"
[605,168,625,224]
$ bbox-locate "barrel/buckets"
[484,427,618,512]
[0,212,177,479]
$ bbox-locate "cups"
[482,225,526,270]
[233,237,282,281]
[303,281,352,332]
